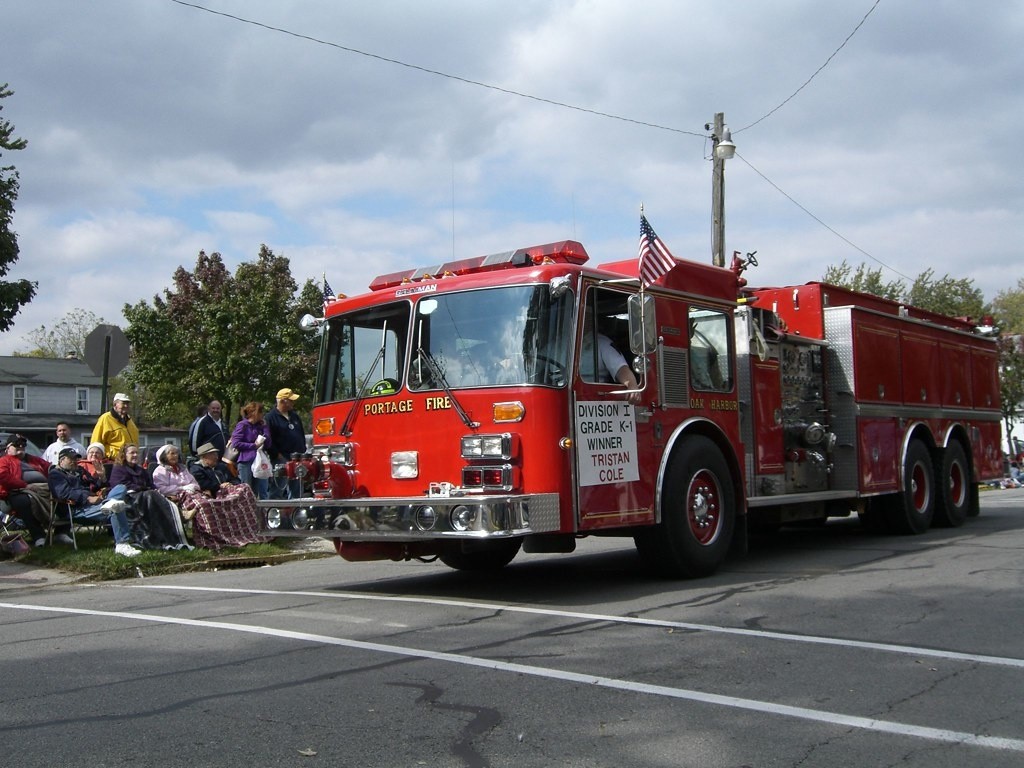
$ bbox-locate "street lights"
[712,127,737,267]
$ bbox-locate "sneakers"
[115,543,142,558]
[101,499,127,515]
[162,542,188,551]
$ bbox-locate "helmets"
[371,379,400,395]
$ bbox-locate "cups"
[255,434,266,446]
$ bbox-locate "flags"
[322,279,336,317]
[638,218,677,292]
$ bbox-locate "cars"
[0,433,50,492]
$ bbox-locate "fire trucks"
[257,240,1003,580]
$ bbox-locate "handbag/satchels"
[223,439,241,465]
[1,533,31,554]
[251,445,274,479]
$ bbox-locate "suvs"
[138,444,183,482]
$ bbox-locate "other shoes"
[35,538,46,547]
[58,534,73,542]
[181,508,196,523]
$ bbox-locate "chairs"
[0,459,198,550]
[599,315,641,383]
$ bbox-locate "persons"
[189,442,258,541]
[47,448,143,558]
[189,400,241,484]
[152,444,223,549]
[78,442,114,499]
[40,421,87,467]
[264,387,307,499]
[90,392,140,462]
[231,402,270,500]
[0,434,74,548]
[109,443,198,551]
[526,296,642,406]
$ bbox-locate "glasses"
[8,443,27,448]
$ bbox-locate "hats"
[86,442,105,457]
[195,442,220,455]
[6,434,27,445]
[113,393,133,402]
[276,388,300,401]
[58,448,83,459]
